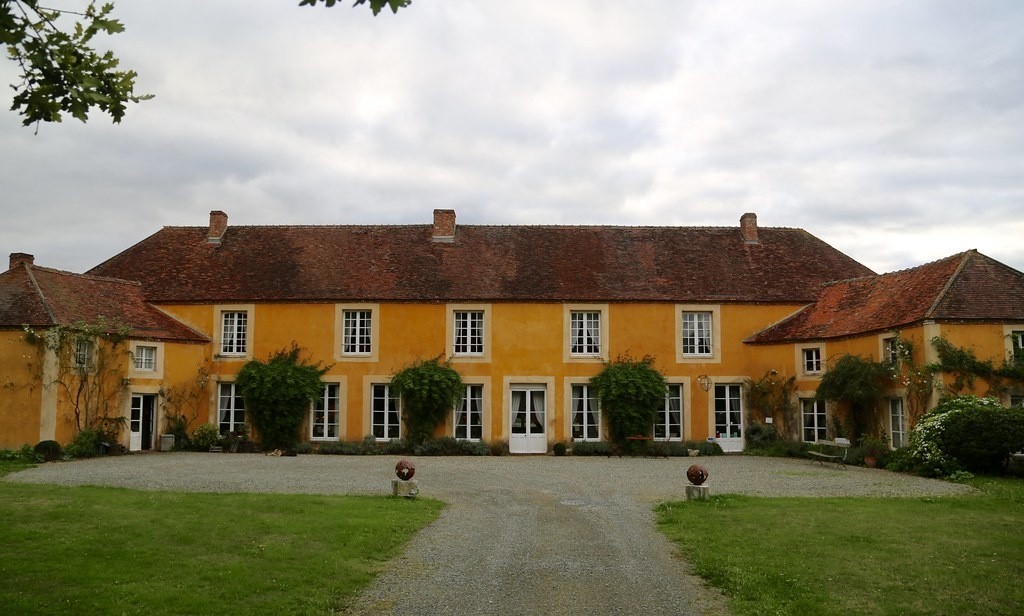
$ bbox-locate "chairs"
[652,435,671,459]
[603,434,622,459]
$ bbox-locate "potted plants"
[238,424,253,453]
[862,437,885,468]
[192,424,222,452]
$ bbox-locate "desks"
[624,437,651,459]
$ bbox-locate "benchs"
[807,439,850,470]
